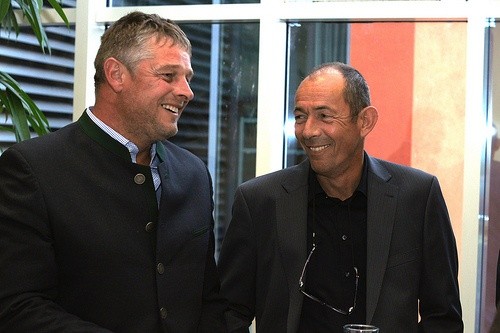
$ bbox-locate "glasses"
[298,244,359,315]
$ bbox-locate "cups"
[342,323,380,333]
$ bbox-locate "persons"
[0,11,217,333]
[216,62,464,333]
